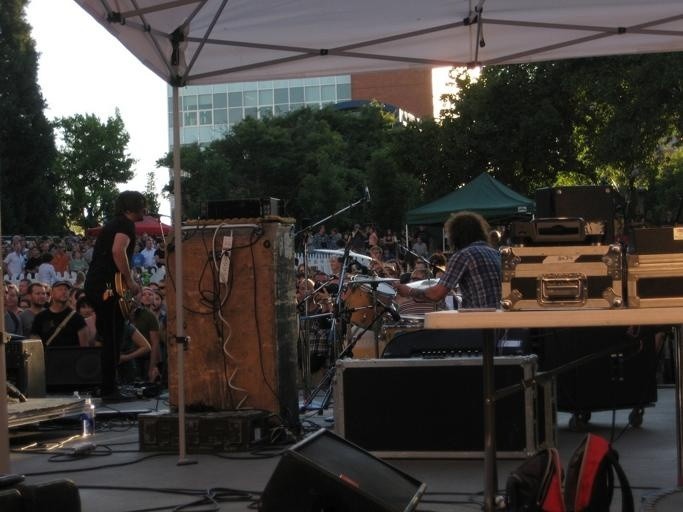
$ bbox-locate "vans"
[512,216,584,244]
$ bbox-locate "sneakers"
[103,390,136,402]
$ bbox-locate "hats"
[53,281,73,288]
[143,288,163,299]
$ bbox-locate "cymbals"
[406,279,440,289]
[314,249,374,261]
[348,275,400,284]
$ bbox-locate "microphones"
[364,187,370,203]
[345,224,360,251]
[146,213,162,219]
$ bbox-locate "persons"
[119,234,169,395]
[293,222,448,373]
[84,191,149,404]
[383,212,504,359]
[0,234,97,349]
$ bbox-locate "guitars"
[114,266,143,319]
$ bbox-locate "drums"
[399,297,445,318]
[345,274,396,331]
[347,320,425,359]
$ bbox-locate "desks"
[423,299,683,511]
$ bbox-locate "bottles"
[81,398,95,438]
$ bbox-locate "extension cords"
[219,236,233,284]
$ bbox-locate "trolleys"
[522,326,658,431]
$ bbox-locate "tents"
[88,215,172,236]
[405,172,537,249]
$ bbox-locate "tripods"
[325,252,349,371]
[304,309,383,416]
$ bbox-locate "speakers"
[5,339,47,398]
[207,199,261,219]
[535,185,615,245]
[257,427,427,512]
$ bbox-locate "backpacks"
[506,431,635,512]
